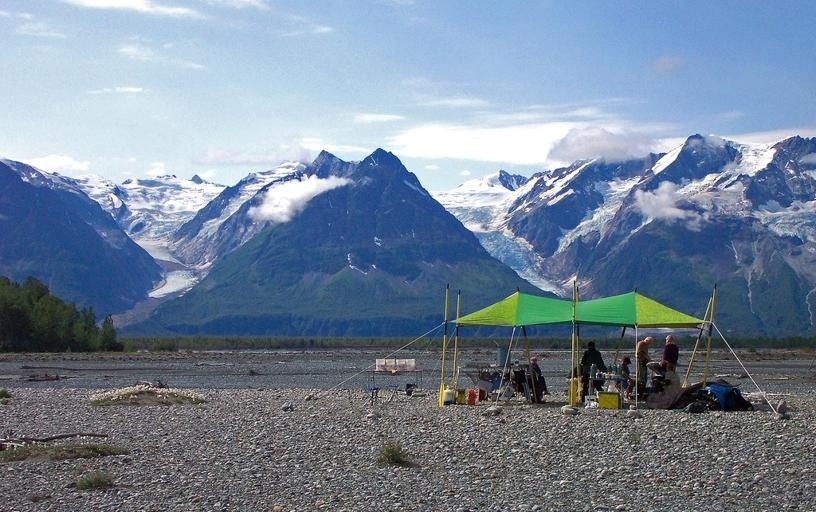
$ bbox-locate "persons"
[614,355,635,404]
[528,357,551,404]
[566,341,607,406]
[634,335,679,402]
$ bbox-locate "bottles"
[589,363,597,378]
[607,362,622,373]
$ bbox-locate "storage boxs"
[599,392,621,409]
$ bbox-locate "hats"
[621,355,632,364]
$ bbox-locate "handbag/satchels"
[682,401,708,413]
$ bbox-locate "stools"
[363,385,401,405]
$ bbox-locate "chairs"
[479,366,511,395]
[514,370,545,404]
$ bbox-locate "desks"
[460,364,531,403]
[587,377,627,409]
[366,369,422,392]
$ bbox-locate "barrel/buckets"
[497,345,511,366]
